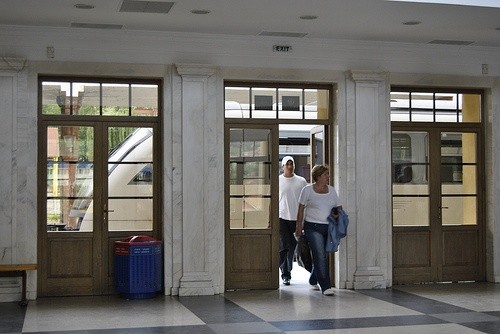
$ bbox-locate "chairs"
[397,168,413,184]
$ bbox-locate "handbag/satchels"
[296,233,312,272]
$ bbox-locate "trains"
[63,93,464,235]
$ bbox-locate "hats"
[282,156,294,166]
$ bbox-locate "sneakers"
[283,279,290,284]
[309,281,320,290]
[323,288,334,295]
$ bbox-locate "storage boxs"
[116,236,164,299]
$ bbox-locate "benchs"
[1,265,38,308]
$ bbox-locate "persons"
[295,165,349,297]
[279,155,308,286]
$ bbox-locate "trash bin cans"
[113,235,163,300]
